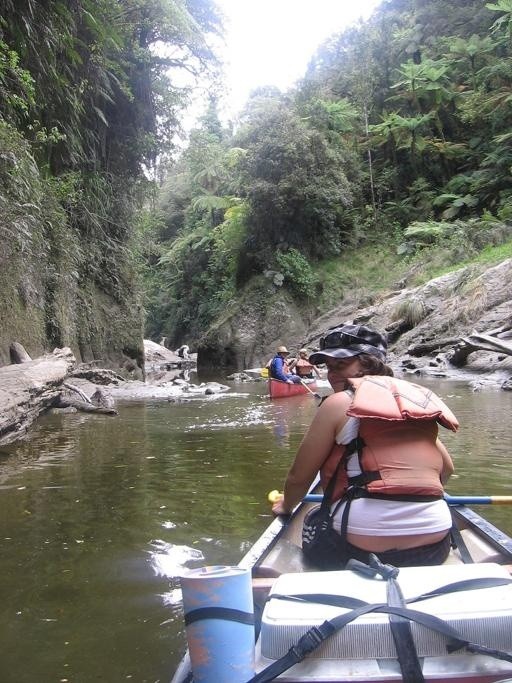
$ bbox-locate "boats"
[268,378,317,398]
[170,469,512,683]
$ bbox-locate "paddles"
[300,380,322,399]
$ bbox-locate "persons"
[272,323,456,570]
[266,345,322,381]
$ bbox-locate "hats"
[308,323,388,366]
[276,345,290,355]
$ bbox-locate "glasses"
[319,331,387,351]
